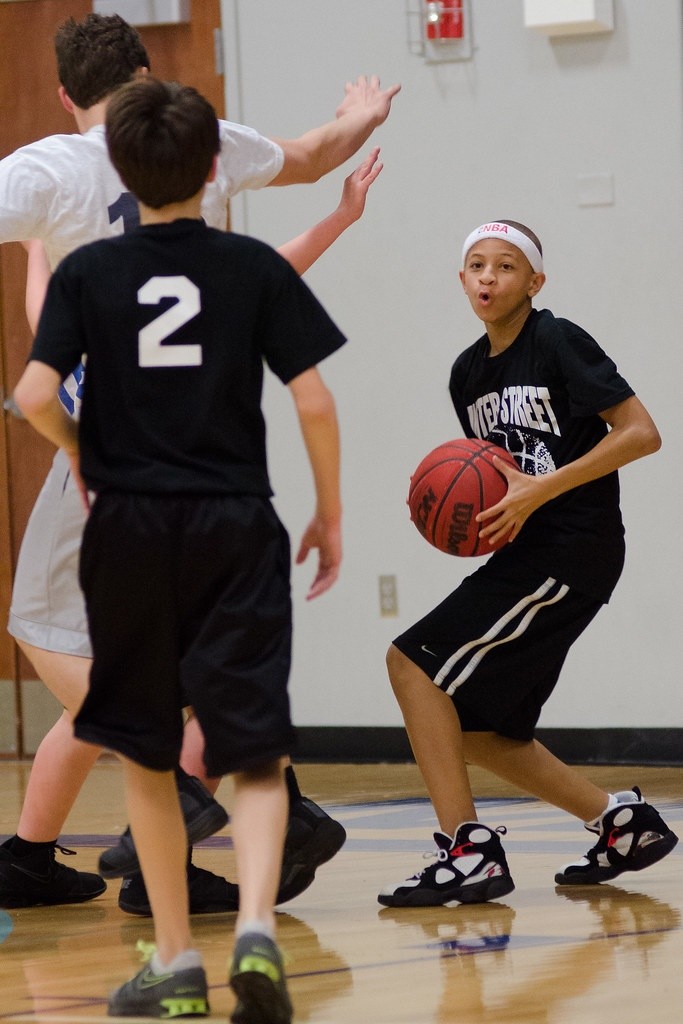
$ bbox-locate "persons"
[0,14,403,915]
[378,220,679,908]
[12,77,347,1024]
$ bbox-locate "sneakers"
[275,797,346,906]
[228,931,292,1024]
[118,851,240,915]
[377,823,516,905]
[555,787,678,884]
[98,776,228,879]
[108,940,207,1018]
[0,836,107,908]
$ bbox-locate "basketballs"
[409,438,522,558]
